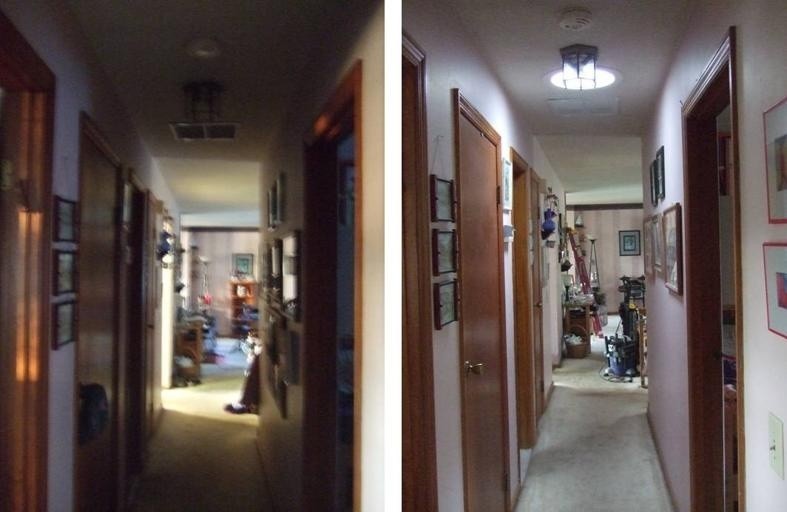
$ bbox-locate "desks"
[564,300,592,354]
[174,321,203,376]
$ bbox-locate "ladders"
[566,227,604,338]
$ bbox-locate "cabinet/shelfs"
[637,311,648,384]
[230,281,257,338]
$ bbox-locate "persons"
[223,351,256,417]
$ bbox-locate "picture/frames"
[429,174,459,330]
[763,242,787,339]
[762,96,787,224]
[619,230,640,256]
[644,146,683,296]
[232,253,253,276]
[53,194,78,350]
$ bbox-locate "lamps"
[180,82,223,121]
[558,43,599,91]
